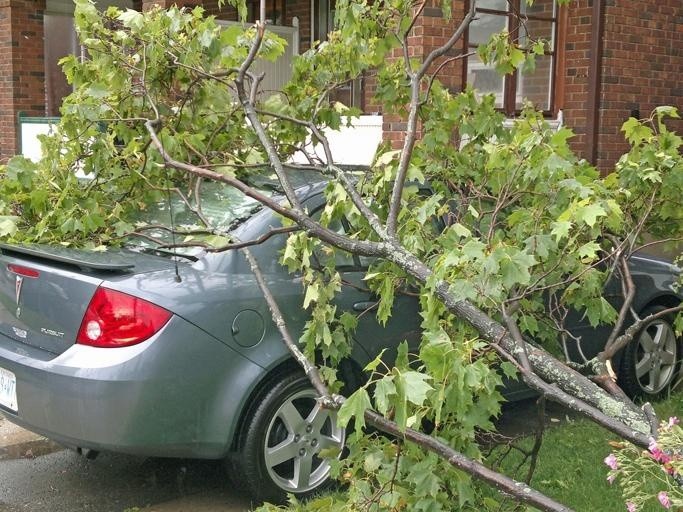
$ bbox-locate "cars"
[2,161,681,504]
